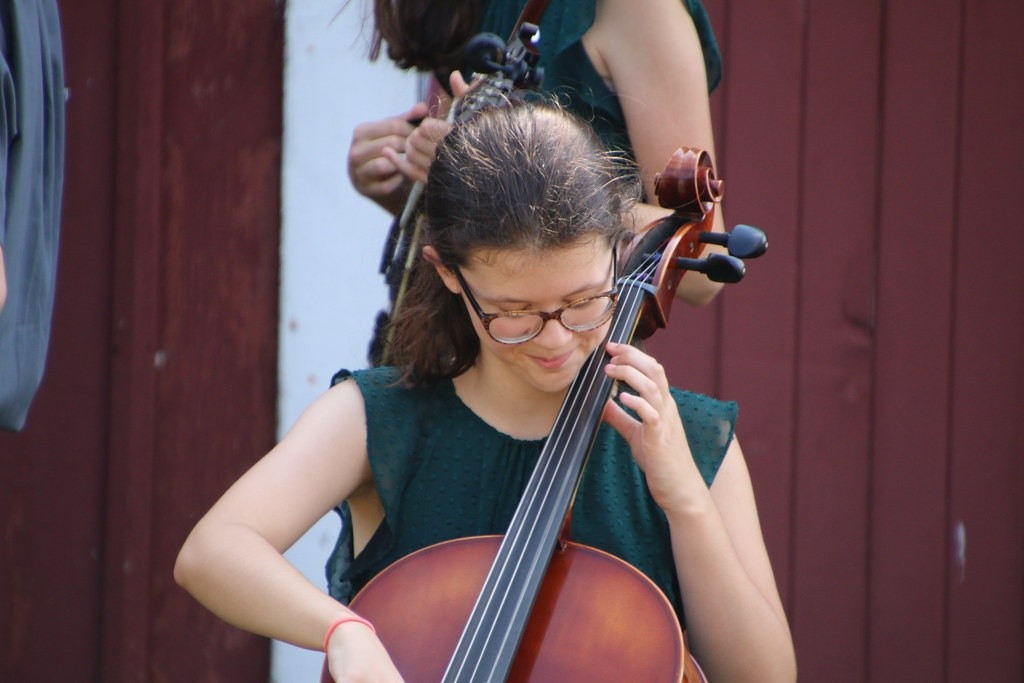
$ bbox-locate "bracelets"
[323,616,378,659]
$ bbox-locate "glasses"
[449,245,622,345]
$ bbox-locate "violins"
[319,144,772,683]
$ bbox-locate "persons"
[172,101,802,683]
[343,0,732,385]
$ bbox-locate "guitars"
[362,17,556,368]
